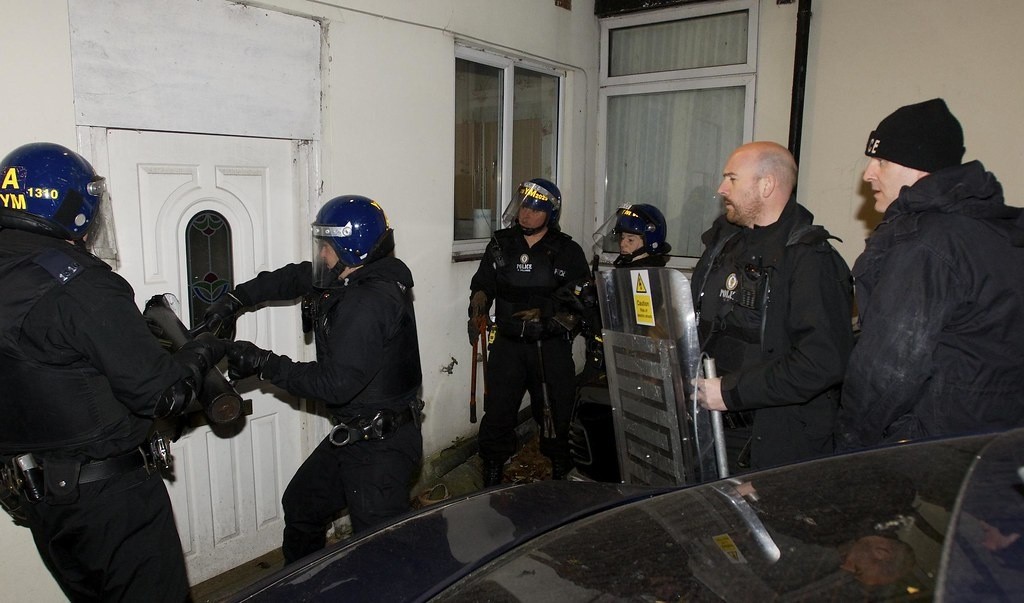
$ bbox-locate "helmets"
[614,203,666,255]
[312,195,388,267]
[518,179,562,230]
[0,143,100,240]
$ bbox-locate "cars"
[215,428,1024,603]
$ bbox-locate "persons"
[0,143,225,603]
[204,195,425,566]
[550,203,684,484]
[244,430,1024,603]
[468,178,591,491]
[835,97,1024,455]
[690,141,854,483]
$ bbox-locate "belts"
[78,445,150,483]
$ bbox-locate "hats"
[865,97,965,175]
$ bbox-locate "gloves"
[181,331,226,373]
[227,340,271,381]
[203,291,244,340]
